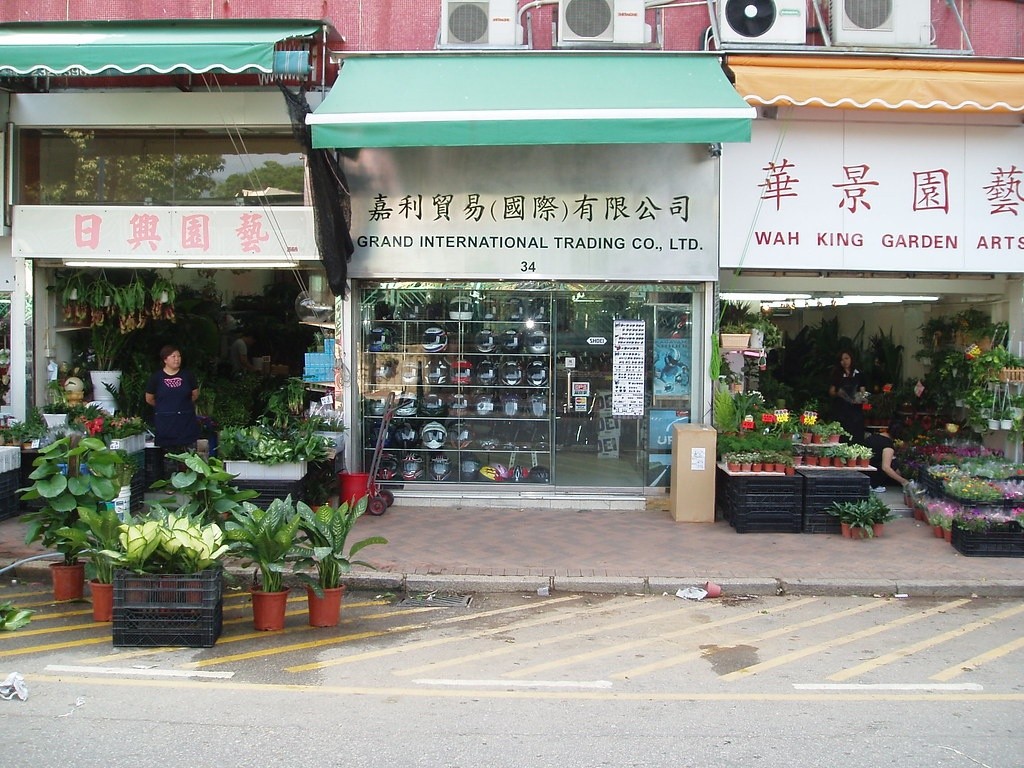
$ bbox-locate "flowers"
[987,479,1024,501]
[939,504,957,531]
[934,438,1004,466]
[997,462,1024,478]
[109,416,149,439]
[84,416,113,443]
[893,433,932,479]
[956,508,1024,533]
[943,467,1002,499]
[927,464,971,479]
[926,501,948,526]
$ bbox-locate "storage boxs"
[1005,498,1024,511]
[801,504,842,534]
[951,519,1024,558]
[127,450,146,489]
[222,459,308,481]
[130,483,144,513]
[228,473,308,511]
[936,483,1005,510]
[669,423,717,524]
[111,564,224,607]
[112,598,224,648]
[0,445,21,473]
[917,466,942,498]
[721,472,805,505]
[145,448,165,493]
[721,493,803,534]
[303,339,335,383]
[315,430,345,455]
[0,468,20,494]
[796,470,871,505]
[0,488,20,522]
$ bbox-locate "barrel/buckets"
[338,472,370,508]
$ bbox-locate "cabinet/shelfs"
[360,287,556,485]
[299,321,335,388]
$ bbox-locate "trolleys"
[366,392,413,516]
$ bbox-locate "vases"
[944,529,952,542]
[932,526,944,538]
[110,432,146,454]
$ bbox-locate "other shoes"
[903,479,929,495]
[164,489,176,495]
[869,485,886,493]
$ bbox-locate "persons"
[830,352,865,446]
[231,334,260,381]
[862,422,910,494]
[146,347,198,493]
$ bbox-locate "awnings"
[0,15,321,75]
[303,56,758,145]
[724,48,1024,116]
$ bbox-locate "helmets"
[368,296,551,483]
[664,347,681,367]
[575,350,594,371]
[556,350,573,371]
[596,351,613,372]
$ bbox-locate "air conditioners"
[441,0,516,46]
[557,0,645,50]
[829,0,931,47]
[716,0,807,44]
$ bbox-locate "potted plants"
[966,344,1024,446]
[61,272,178,334]
[222,493,296,631]
[864,376,937,426]
[902,480,915,505]
[52,506,124,622]
[718,444,876,477]
[39,380,77,426]
[868,496,897,537]
[0,421,46,450]
[713,381,853,443]
[12,429,125,602]
[720,320,751,348]
[903,485,921,508]
[912,495,925,521]
[98,503,228,604]
[749,315,781,348]
[844,500,875,539]
[823,501,858,538]
[296,493,388,628]
[89,325,132,401]
[303,476,334,512]
